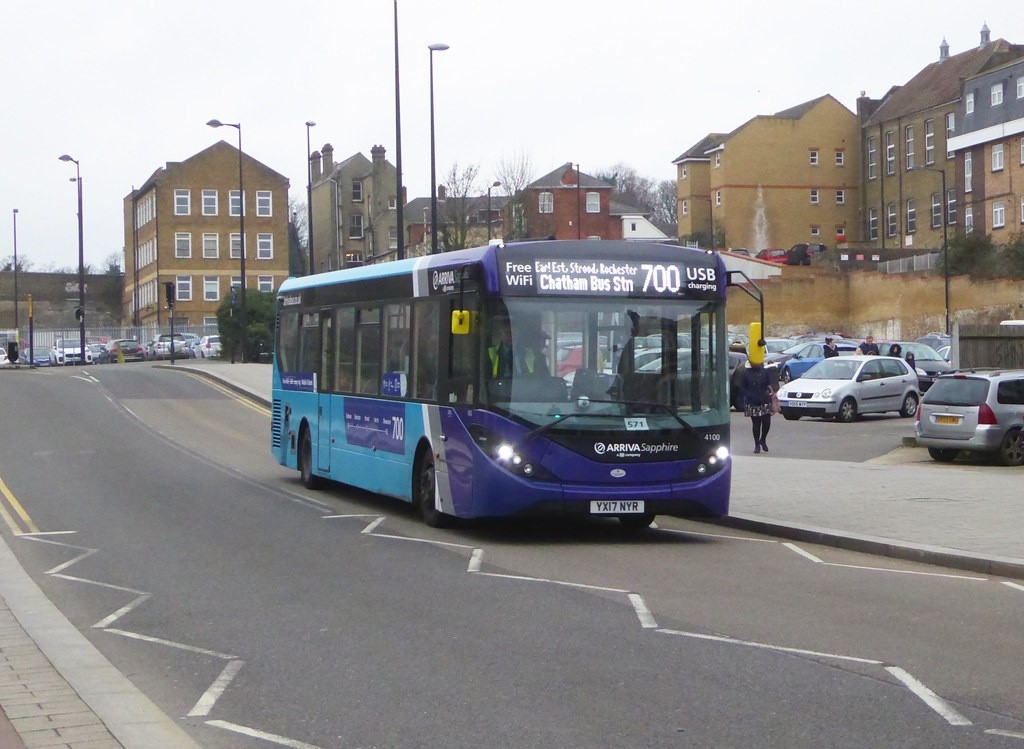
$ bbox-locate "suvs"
[913,366,1024,467]
[784,242,828,266]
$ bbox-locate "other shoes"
[760,440,768,451]
[754,446,760,453]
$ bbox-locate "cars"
[755,247,786,264]
[876,334,952,392]
[729,248,752,258]
[776,355,920,423]
[0,332,223,368]
[547,323,876,409]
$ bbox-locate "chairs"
[569,368,623,401]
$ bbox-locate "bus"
[271,235,769,530]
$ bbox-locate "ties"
[508,350,517,376]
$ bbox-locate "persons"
[488,324,550,377]
[860,335,879,356]
[887,344,901,358]
[905,351,915,371]
[739,361,773,453]
[823,337,839,358]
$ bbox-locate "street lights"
[13,208,19,343]
[428,43,451,254]
[937,169,949,336]
[488,181,501,241]
[58,154,86,365]
[205,119,246,310]
[69,177,83,234]
[306,121,317,274]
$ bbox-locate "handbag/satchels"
[769,385,780,412]
